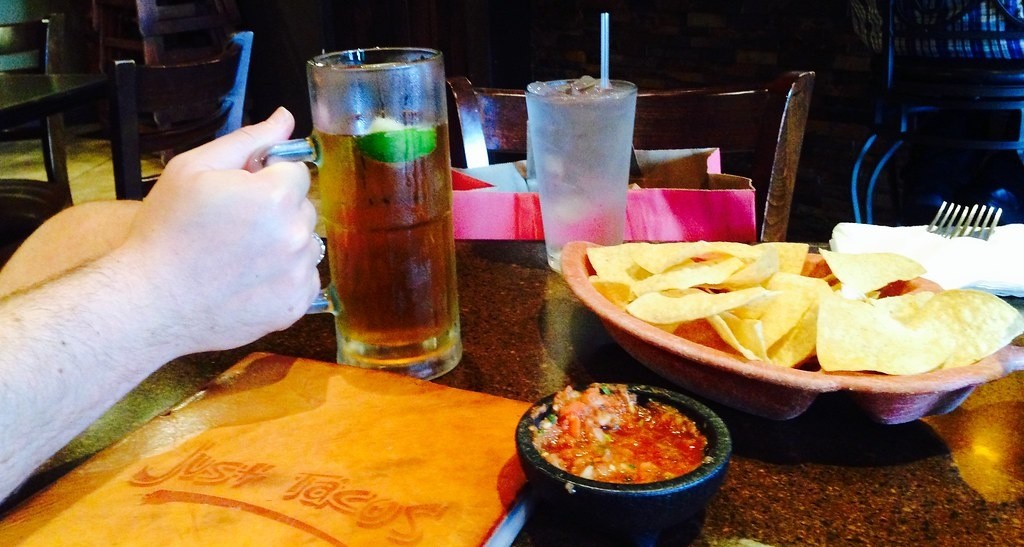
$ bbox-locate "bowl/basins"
[515,383,731,547]
[562,237,1023,422]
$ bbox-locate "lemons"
[356,125,438,164]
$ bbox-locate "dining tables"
[0,239,1024,547]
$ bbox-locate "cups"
[523,78,637,274]
[246,45,465,381]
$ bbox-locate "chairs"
[0,0,256,199]
[445,68,816,241]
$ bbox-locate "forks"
[925,200,971,239]
[957,204,1003,240]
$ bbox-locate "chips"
[586,239,1024,374]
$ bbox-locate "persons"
[849,0,1024,225]
[0,106,321,505]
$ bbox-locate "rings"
[312,232,326,265]
